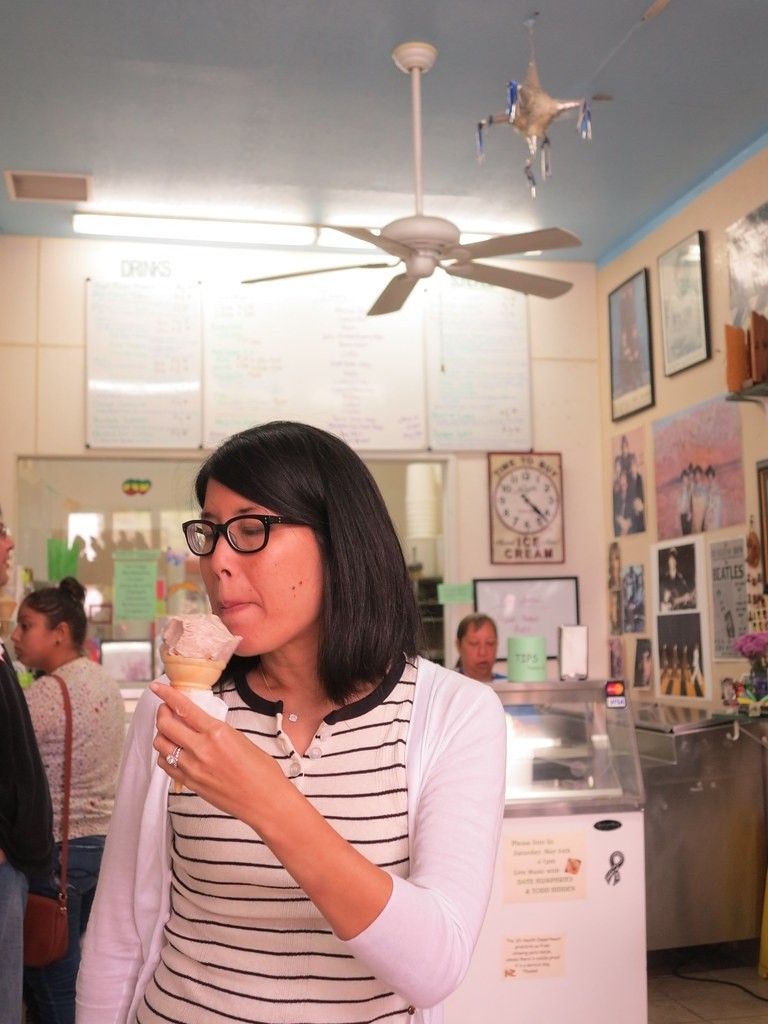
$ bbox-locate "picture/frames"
[606,267,657,422]
[657,230,711,377]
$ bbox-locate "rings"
[165,746,182,767]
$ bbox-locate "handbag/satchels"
[23,894,69,968]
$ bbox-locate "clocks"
[487,449,566,565]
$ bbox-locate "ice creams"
[158,611,243,692]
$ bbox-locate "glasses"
[182,515,315,556]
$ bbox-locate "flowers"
[730,630,768,689]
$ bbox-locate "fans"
[239,40,586,319]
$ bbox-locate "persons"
[633,638,653,687]
[0,506,53,1024]
[622,565,644,633]
[611,434,646,537]
[9,576,126,1024]
[609,590,622,636]
[74,421,507,1024]
[607,541,621,592]
[676,461,722,536]
[452,612,544,737]
[662,547,688,605]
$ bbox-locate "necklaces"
[258,662,327,723]
